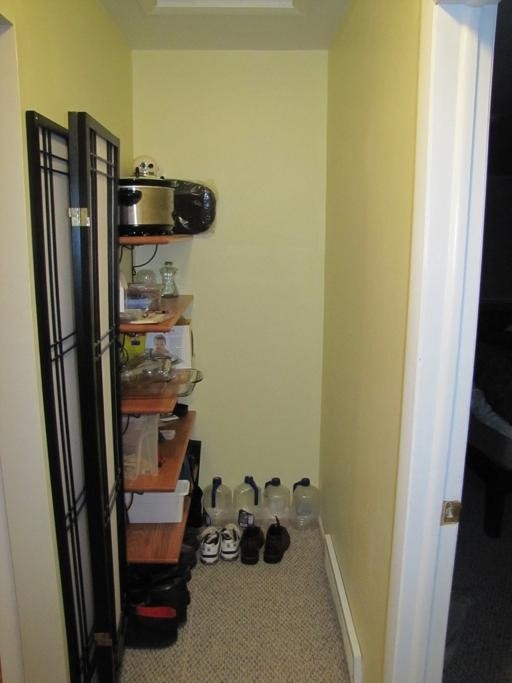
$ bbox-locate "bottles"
[204,476,319,534]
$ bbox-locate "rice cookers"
[117,174,176,232]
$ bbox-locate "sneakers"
[221,522,242,561]
[126,524,200,628]
[263,522,291,564]
[200,526,221,567]
[239,524,265,566]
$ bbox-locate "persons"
[153,335,173,354]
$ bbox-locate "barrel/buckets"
[202,477,319,529]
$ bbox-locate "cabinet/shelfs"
[117,234,204,564]
